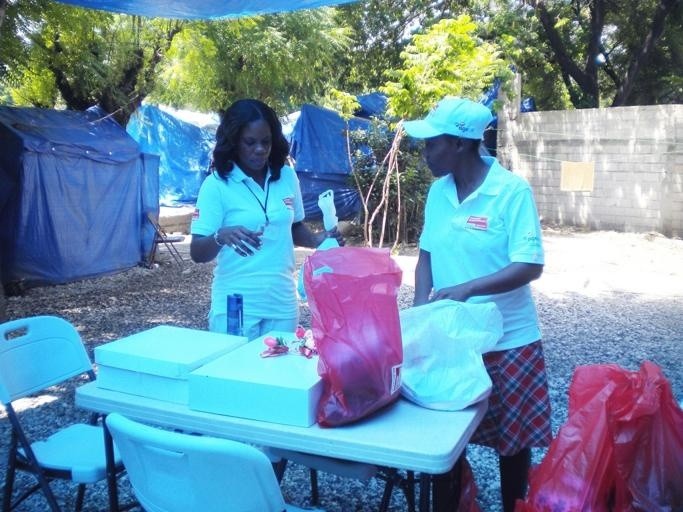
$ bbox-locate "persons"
[190,99,344,346]
[403,98,553,511]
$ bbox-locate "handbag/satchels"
[515,360,683,512]
[303,247,405,426]
[396,299,503,411]
[451,456,481,512]
[298,189,341,300]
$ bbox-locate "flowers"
[259,325,315,359]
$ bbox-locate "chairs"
[0,311,129,511]
[106,409,322,512]
[145,213,189,267]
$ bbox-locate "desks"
[74,379,492,511]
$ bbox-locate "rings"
[231,244,236,249]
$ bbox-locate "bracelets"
[213,233,222,246]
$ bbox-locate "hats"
[400,98,494,139]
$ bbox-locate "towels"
[558,159,597,193]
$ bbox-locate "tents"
[281,93,426,222]
[126,106,216,209]
[482,81,538,130]
[0,108,159,284]
